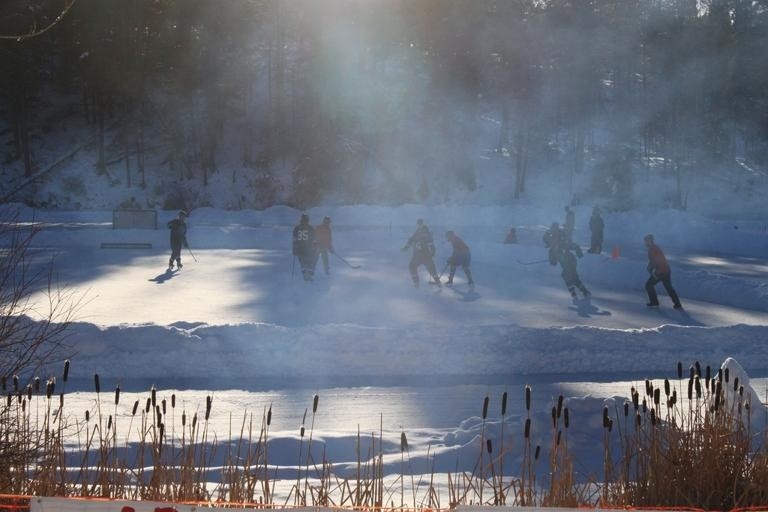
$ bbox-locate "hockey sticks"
[516,258,549,265]
[429,262,449,285]
[332,252,363,269]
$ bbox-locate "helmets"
[646,234,653,246]
[179,211,184,217]
[300,214,309,224]
[322,216,331,226]
[444,231,455,241]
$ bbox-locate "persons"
[642,233,682,309]
[444,230,473,285]
[543,194,604,255]
[168,209,189,268]
[292,213,316,281]
[313,216,334,276]
[416,218,436,256]
[504,228,518,244]
[400,223,442,289]
[548,234,591,306]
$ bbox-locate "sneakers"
[646,301,658,306]
[169,262,175,266]
[672,302,682,309]
[177,262,184,265]
[413,280,421,286]
[583,290,591,295]
[436,281,443,288]
[445,280,454,285]
[573,295,576,302]
[325,270,330,274]
[304,271,314,280]
[467,279,471,283]
